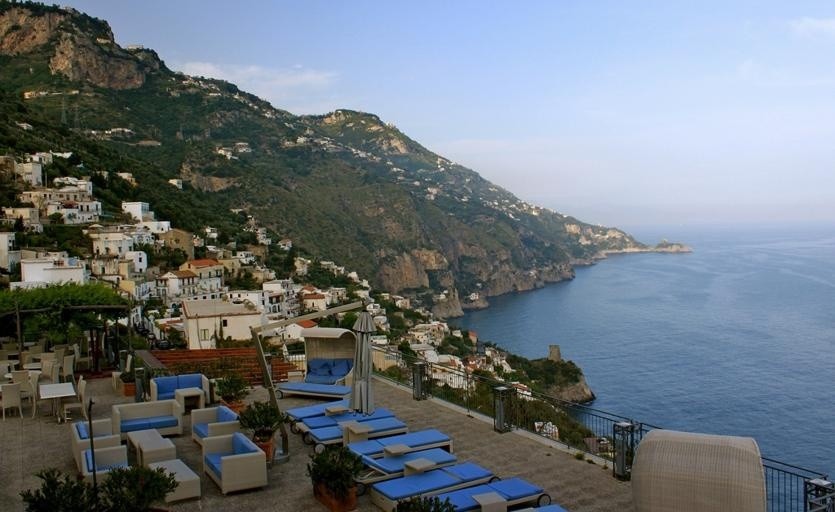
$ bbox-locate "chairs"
[288,329,358,389]
[0,329,133,423]
[274,381,568,512]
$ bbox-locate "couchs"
[71,373,269,508]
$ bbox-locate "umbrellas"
[350,309,378,419]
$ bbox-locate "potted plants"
[236,400,290,465]
